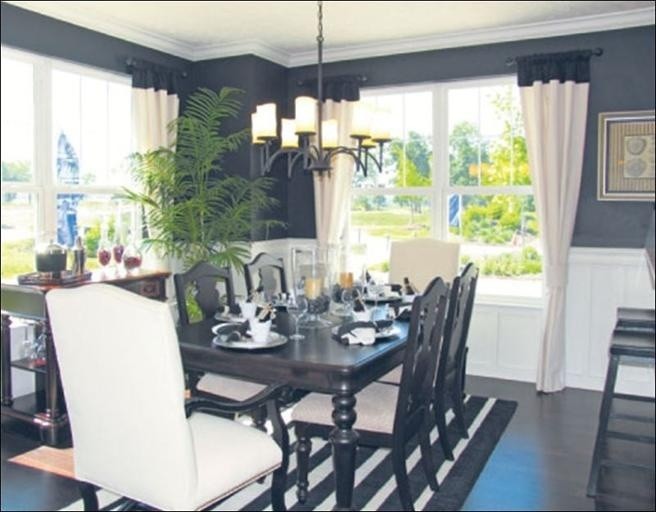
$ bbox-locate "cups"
[250,317,271,343]
[331,244,368,316]
[290,243,332,329]
[403,293,416,303]
[352,310,371,321]
[240,302,256,319]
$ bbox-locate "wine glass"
[286,295,308,341]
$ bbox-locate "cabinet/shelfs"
[465,299,542,384]
[1,267,171,450]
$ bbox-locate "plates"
[331,321,401,340]
[214,309,276,321]
[211,322,277,335]
[213,330,289,350]
[362,292,402,301]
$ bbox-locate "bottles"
[72,235,85,276]
[22,333,46,365]
[97,226,111,270]
[122,234,142,271]
[112,234,123,264]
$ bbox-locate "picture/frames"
[594,107,656,203]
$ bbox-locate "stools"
[583,328,656,503]
[613,304,655,331]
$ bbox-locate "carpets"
[6,386,196,484]
[57,381,520,512]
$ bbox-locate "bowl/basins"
[36,236,67,279]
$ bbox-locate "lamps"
[247,2,394,182]
